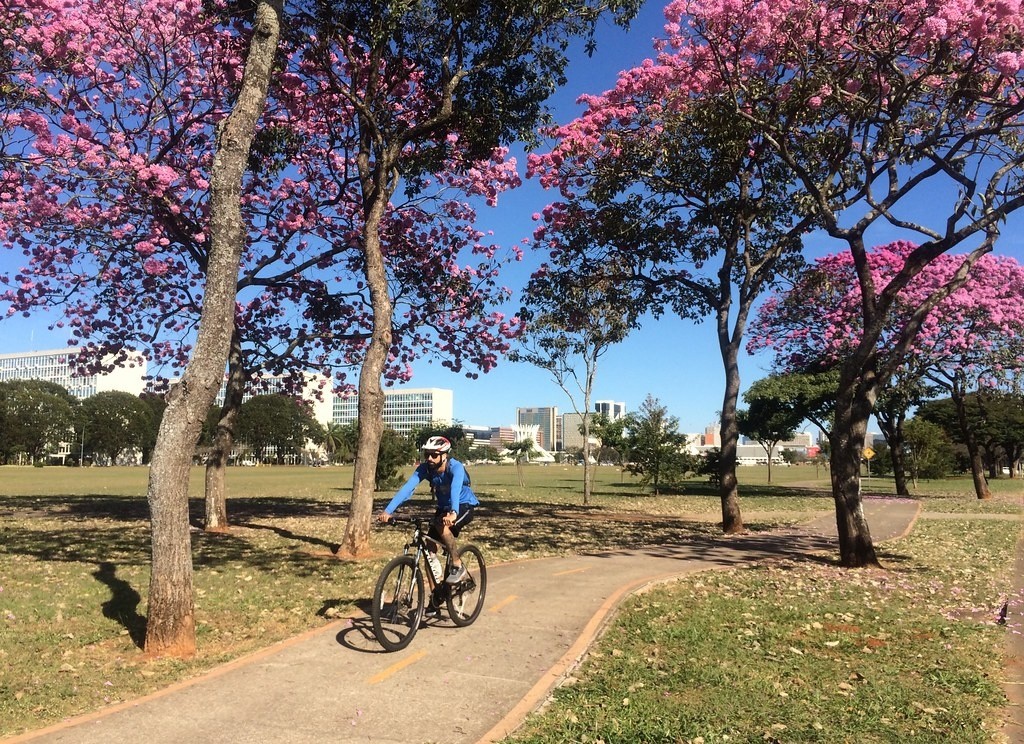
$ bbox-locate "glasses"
[424,452,446,459]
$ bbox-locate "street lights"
[80,421,93,466]
[245,429,254,466]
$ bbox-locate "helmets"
[422,436,451,465]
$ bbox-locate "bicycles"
[370,514,488,651]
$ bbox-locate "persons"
[376,436,479,613]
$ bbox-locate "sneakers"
[445,564,467,584]
[425,594,439,616]
